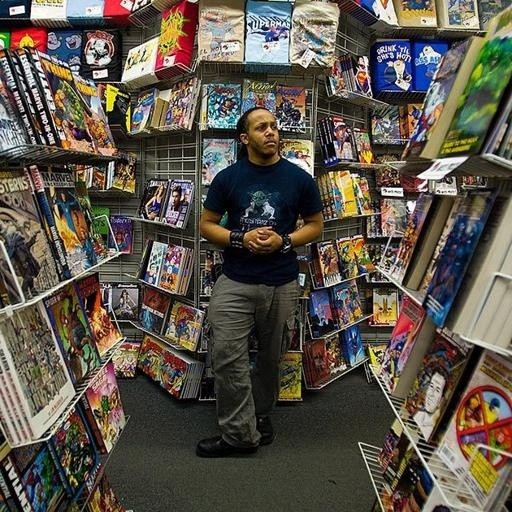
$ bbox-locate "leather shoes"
[196,436,259,458]
[255,415,274,446]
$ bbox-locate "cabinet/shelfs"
[358,146,512,511]
[114,3,379,408]
[0,141,138,512]
[0,13,143,346]
[356,17,484,350]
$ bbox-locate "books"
[0,0,512,512]
